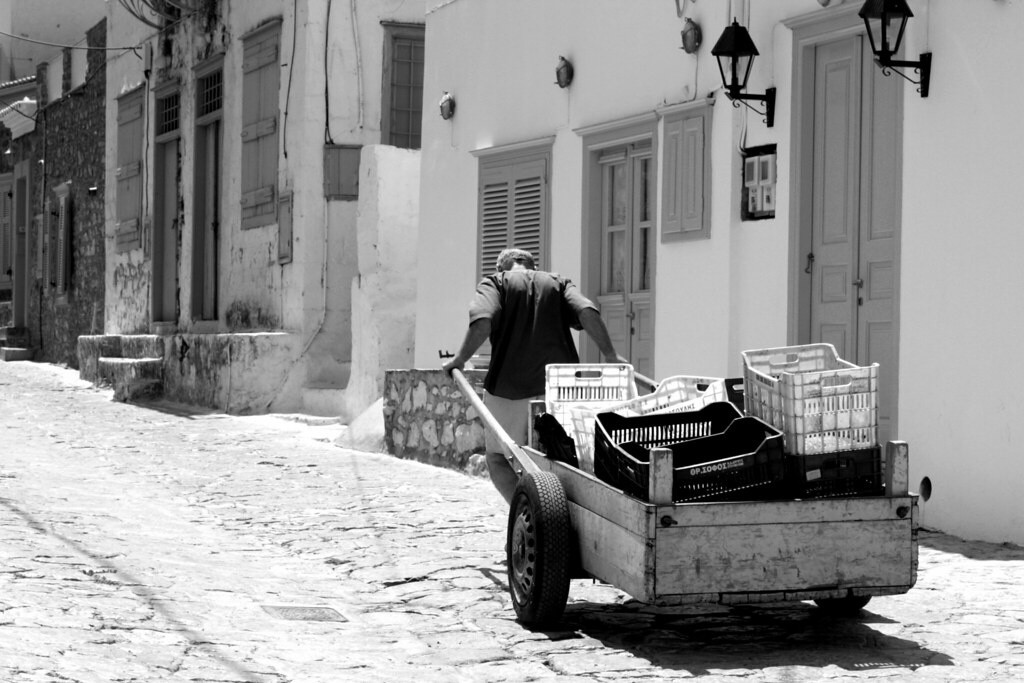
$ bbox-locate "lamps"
[858,0,932,97]
[711,17,777,128]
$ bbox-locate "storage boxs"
[593,401,744,489]
[612,415,784,503]
[545,363,638,436]
[784,444,882,500]
[569,374,729,478]
[651,377,744,413]
[741,343,881,454]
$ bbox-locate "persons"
[443,248,629,505]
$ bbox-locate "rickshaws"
[437,347,921,633]
